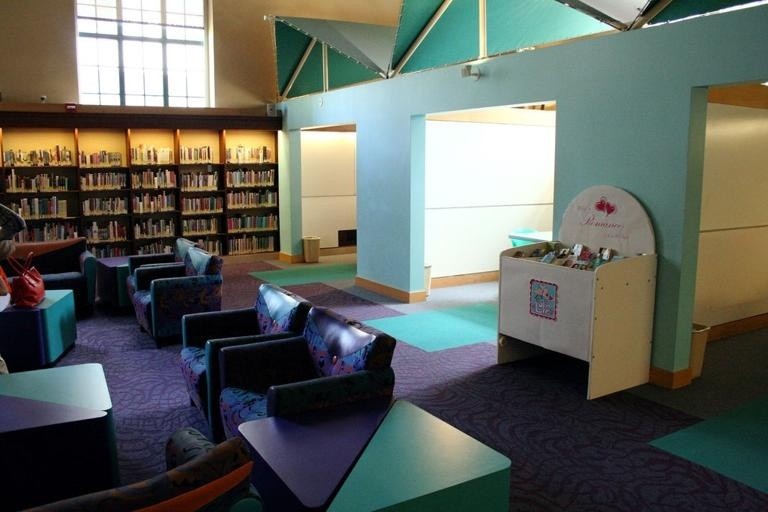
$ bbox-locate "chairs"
[23,427,255,512]
[218,305,397,441]
[179,283,313,445]
[133,249,224,347]
[509,227,538,246]
[127,238,204,307]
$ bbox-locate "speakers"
[266,103,277,117]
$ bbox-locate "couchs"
[0,235,98,316]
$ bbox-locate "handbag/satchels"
[9,266,45,307]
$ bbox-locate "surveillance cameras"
[460,65,480,81]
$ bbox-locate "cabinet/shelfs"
[0,99,282,260]
[491,181,658,404]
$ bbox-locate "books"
[4,143,278,260]
[513,242,649,272]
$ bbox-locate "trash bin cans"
[302,235,320,263]
[689,323,711,379]
[425,263,432,297]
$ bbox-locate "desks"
[94,254,169,312]
[507,228,551,244]
[1,289,78,366]
[0,361,119,512]
[236,398,513,512]
